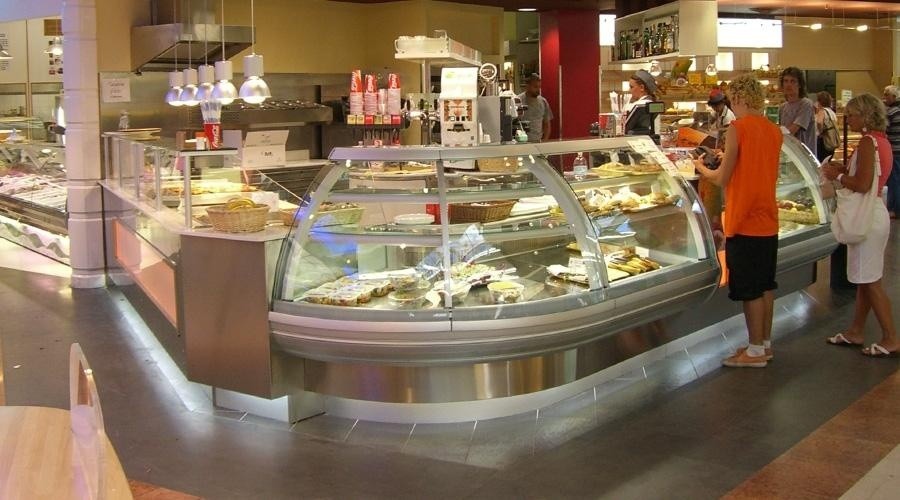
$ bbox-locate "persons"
[516,73,553,143]
[813,91,840,164]
[881,85,900,221]
[706,88,736,136]
[820,93,900,358]
[778,67,818,159]
[615,68,660,164]
[691,77,784,368]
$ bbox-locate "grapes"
[796,195,813,206]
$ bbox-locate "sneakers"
[722,347,773,368]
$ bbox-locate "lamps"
[159,1,273,110]
[780,3,900,34]
[36,16,64,56]
[0,42,15,59]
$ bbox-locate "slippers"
[827,333,900,358]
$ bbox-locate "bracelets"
[835,173,845,183]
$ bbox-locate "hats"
[708,89,726,104]
[634,69,656,92]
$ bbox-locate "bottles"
[618,12,679,59]
[572,152,587,181]
[117,111,129,129]
[406,94,439,113]
[590,121,600,135]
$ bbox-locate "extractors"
[130,0,256,72]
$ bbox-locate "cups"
[349,68,402,116]
[195,132,205,150]
[204,122,221,148]
[174,130,186,146]
[393,34,425,55]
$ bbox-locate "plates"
[393,214,434,224]
[628,204,658,211]
[388,291,424,302]
[591,167,631,177]
[102,127,163,137]
[632,171,658,176]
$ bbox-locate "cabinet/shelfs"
[614,0,718,63]
[98,124,301,422]
[648,121,841,371]
[268,130,722,428]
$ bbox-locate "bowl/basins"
[438,282,471,303]
[487,282,524,303]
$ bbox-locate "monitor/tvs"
[624,104,658,134]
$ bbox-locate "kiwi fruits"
[795,205,806,211]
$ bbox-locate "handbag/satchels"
[822,120,841,150]
[830,189,876,244]
